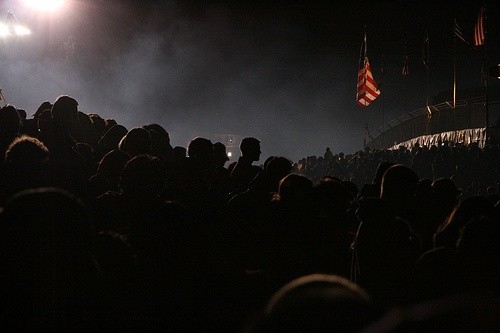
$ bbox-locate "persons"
[1,92,500,333]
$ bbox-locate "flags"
[400,44,409,76]
[472,4,487,49]
[421,33,434,72]
[355,35,381,109]
[454,17,472,46]
